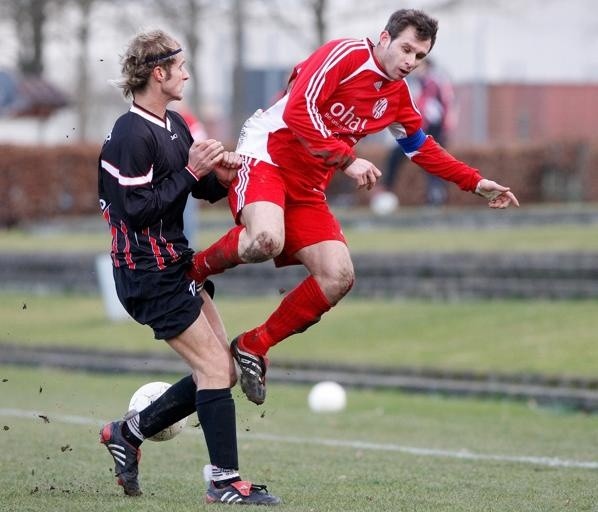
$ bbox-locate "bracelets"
[217,179,230,189]
[185,164,200,183]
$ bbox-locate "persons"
[180,7,522,405]
[383,57,466,205]
[96,29,283,506]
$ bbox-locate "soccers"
[307,382,346,412]
[128,382,189,441]
[368,190,397,217]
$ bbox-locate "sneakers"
[229,332,269,406]
[206,478,281,505]
[98,419,142,496]
